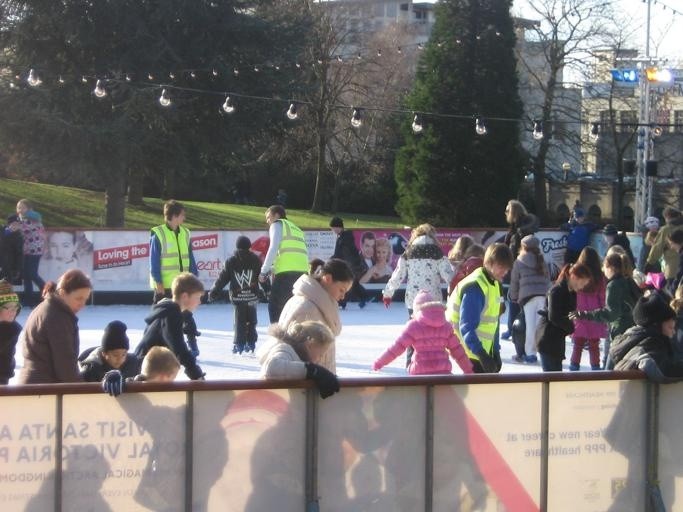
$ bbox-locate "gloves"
[208,292,217,302]
[478,350,497,373]
[265,285,271,293]
[383,296,391,308]
[305,362,340,399]
[568,310,588,321]
[103,370,123,396]
[493,352,501,371]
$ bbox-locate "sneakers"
[512,352,537,362]
[338,301,346,307]
[244,344,256,351]
[189,350,199,356]
[232,345,243,352]
[359,295,368,306]
[502,331,510,337]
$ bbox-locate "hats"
[412,289,433,304]
[102,321,129,349]
[330,217,343,227]
[236,236,250,250]
[0,280,19,306]
[522,235,539,247]
[575,209,584,217]
[646,217,659,228]
[632,295,675,326]
[669,230,682,243]
[603,224,617,235]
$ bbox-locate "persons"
[135,271,206,381]
[264,321,341,396]
[259,204,309,323]
[277,258,359,375]
[41,230,79,285]
[383,223,457,369]
[357,232,393,283]
[103,345,181,396]
[5,198,46,304]
[373,289,474,389]
[80,319,142,382]
[0,217,24,284]
[208,236,271,354]
[447,200,682,383]
[327,216,370,310]
[13,269,93,383]
[0,278,22,385]
[147,200,201,354]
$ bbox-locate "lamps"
[221,94,236,114]
[474,115,486,135]
[412,112,423,133]
[532,119,545,140]
[157,85,172,108]
[94,79,107,98]
[286,99,299,121]
[350,106,362,128]
